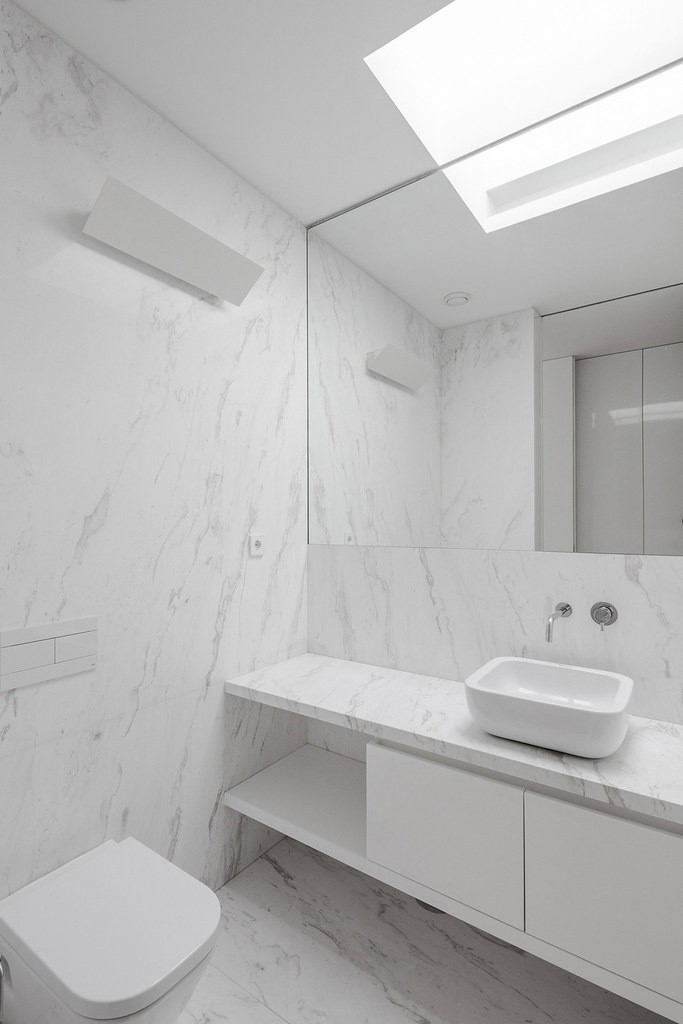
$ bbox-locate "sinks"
[464,656,634,760]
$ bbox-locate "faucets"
[544,602,573,643]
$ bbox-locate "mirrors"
[307,61,683,556]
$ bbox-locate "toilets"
[0,834,222,1024]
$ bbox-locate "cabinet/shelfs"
[223,653,683,1024]
[576,342,683,556]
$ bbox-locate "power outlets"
[249,535,266,555]
